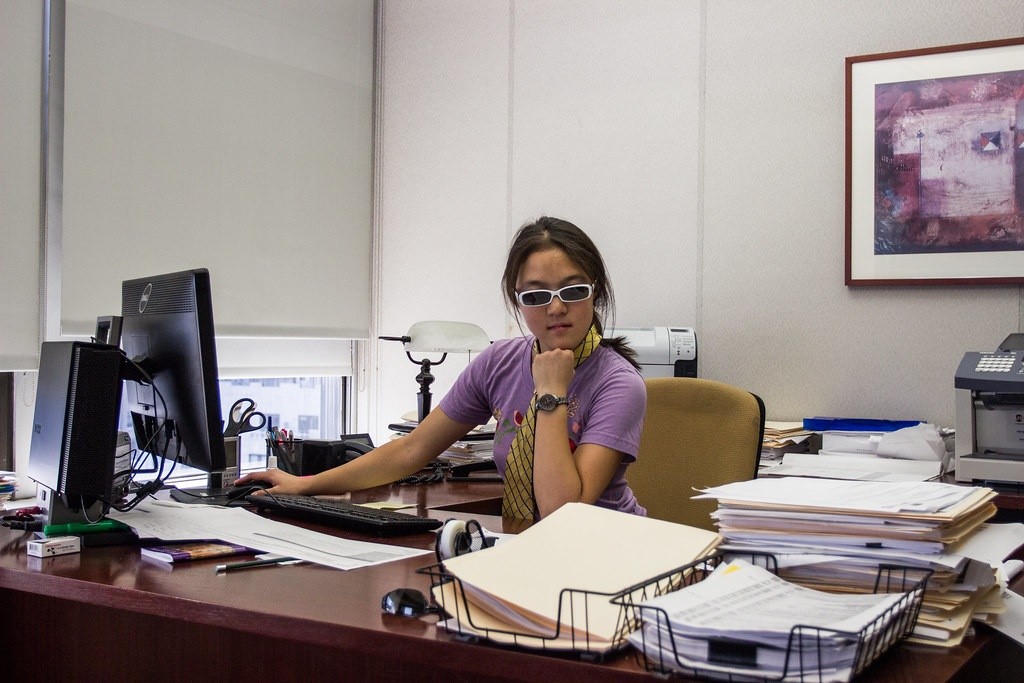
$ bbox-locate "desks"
[1,462,1024,683]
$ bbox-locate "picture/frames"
[843,37,1023,287]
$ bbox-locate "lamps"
[379,321,492,473]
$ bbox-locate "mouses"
[227,481,271,500]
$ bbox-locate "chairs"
[625,377,767,533]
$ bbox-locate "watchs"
[536,391,569,413]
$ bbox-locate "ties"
[501,323,604,520]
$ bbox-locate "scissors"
[223,398,266,437]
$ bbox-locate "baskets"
[610,547,935,683]
[414,527,723,665]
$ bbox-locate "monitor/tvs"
[121,268,228,473]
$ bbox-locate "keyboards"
[243,494,444,537]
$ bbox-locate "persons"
[231,216,651,535]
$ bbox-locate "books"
[140,539,257,563]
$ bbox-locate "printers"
[954,332,1024,483]
[604,327,699,379]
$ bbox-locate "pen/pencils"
[216,558,298,572]
[267,416,299,476]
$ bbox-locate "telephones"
[343,441,373,454]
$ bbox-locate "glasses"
[512,278,598,307]
